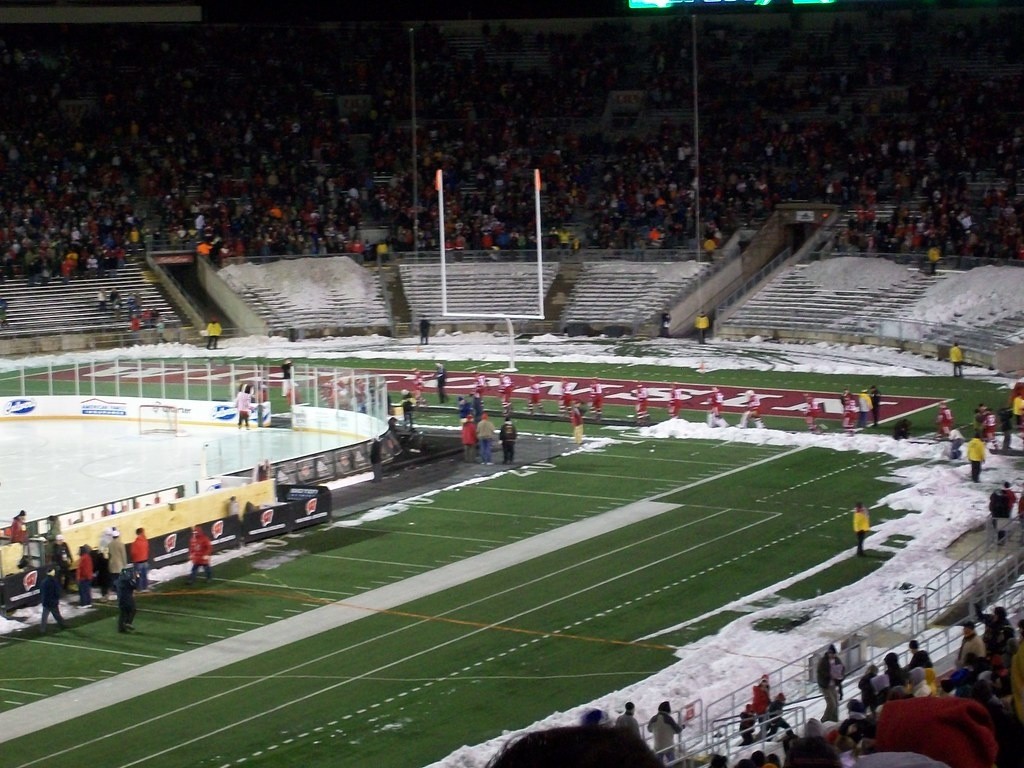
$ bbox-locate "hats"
[467,414,474,419]
[759,674,770,686]
[112,531,120,537]
[625,702,635,710]
[55,535,63,541]
[806,718,821,736]
[658,701,671,713]
[192,528,201,534]
[994,606,1007,617]
[481,412,488,418]
[961,621,975,630]
[828,644,836,653]
[746,704,756,713]
[776,693,785,701]
[909,640,918,649]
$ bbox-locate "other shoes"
[481,462,495,465]
[134,587,150,593]
[117,623,136,634]
[76,603,92,609]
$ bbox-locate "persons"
[950,342,964,377]
[481,700,686,768]
[420,315,431,345]
[0,494,254,636]
[1,17,1024,288]
[96,289,168,345]
[364,363,603,484]
[278,356,296,398]
[853,501,871,557]
[659,306,713,347]
[205,317,222,349]
[933,379,1024,547]
[706,604,1024,768]
[232,380,254,432]
[629,378,914,447]
